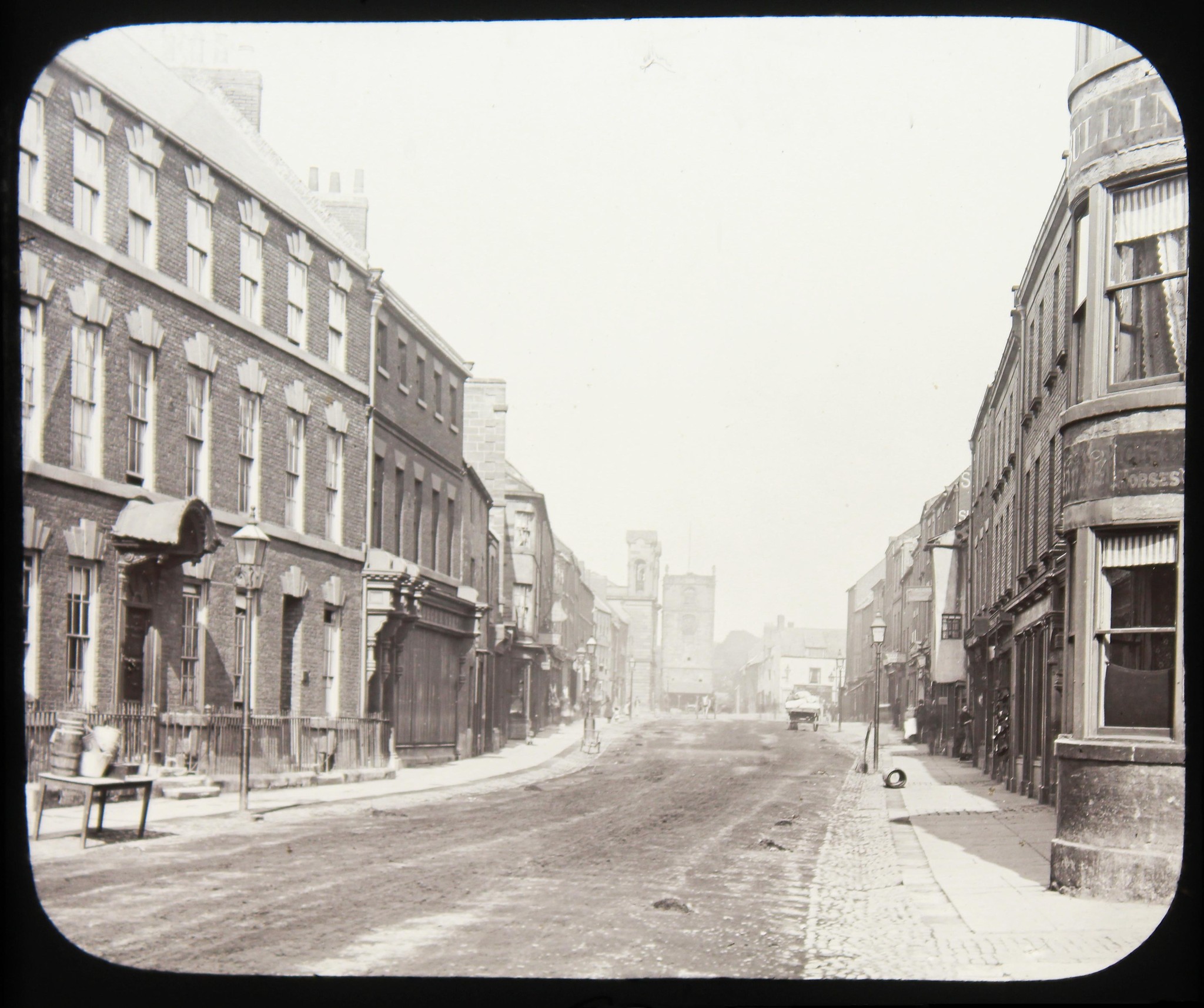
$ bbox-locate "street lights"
[575,636,597,754]
[869,612,887,772]
[231,504,271,811]
[835,649,846,733]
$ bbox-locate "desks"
[36,771,155,848]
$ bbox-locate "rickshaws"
[788,694,822,731]
